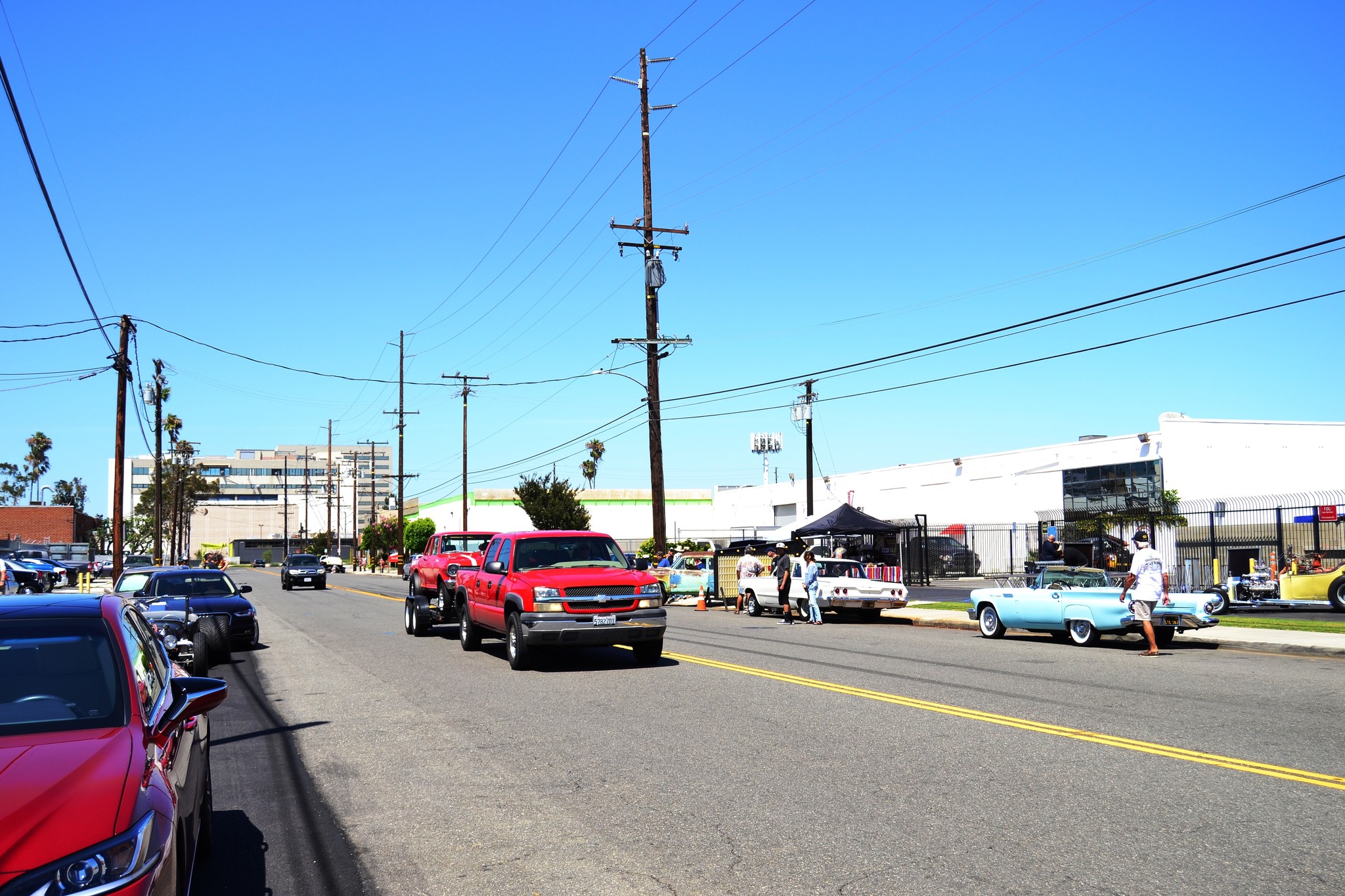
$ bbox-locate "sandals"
[804,620,815,624]
[813,621,823,625]
[734,610,739,614]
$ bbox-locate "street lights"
[1063,494,1075,543]
[259,524,264,539]
[591,370,667,550]
[142,373,201,565]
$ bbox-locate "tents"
[795,503,904,584]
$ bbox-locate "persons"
[802,551,823,625]
[693,559,713,570]
[0,558,7,596]
[175,556,188,566]
[656,552,670,569]
[641,554,654,569]
[831,542,848,577]
[362,555,366,571]
[1119,531,1170,657]
[1041,534,1063,562]
[776,542,795,625]
[767,547,779,576]
[734,546,765,615]
[93,562,102,579]
[204,552,230,572]
[379,559,384,573]
[330,565,339,574]
[664,545,692,599]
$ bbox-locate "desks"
[993,573,1042,587]
[1107,571,1130,588]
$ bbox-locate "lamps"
[953,457,962,466]
[1138,433,1150,442]
[823,476,830,482]
[789,473,795,481]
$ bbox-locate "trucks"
[319,554,346,573]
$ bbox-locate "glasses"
[581,547,591,550]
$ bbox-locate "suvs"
[281,553,326,591]
[402,554,423,581]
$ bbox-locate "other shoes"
[1140,650,1159,658]
[680,597,684,600]
[766,611,776,614]
[791,620,795,625]
[687,595,694,598]
[674,598,677,601]
[777,620,791,625]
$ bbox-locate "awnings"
[939,524,964,534]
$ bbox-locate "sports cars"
[1202,558,1345,613]
[126,594,229,677]
[967,564,1219,647]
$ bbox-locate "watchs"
[1,580,4,582]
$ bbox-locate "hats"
[642,554,650,558]
[683,546,692,551]
[669,547,674,551]
[183,556,188,560]
[578,540,591,549]
[764,547,776,552]
[1131,531,1149,542]
[674,546,684,552]
[775,543,785,548]
[177,557,184,562]
[841,541,847,546]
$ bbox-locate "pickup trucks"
[897,536,980,578]
[404,531,508,639]
[252,559,266,568]
[1076,537,1129,572]
[454,530,667,670]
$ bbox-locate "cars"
[133,569,259,649]
[110,566,191,599]
[640,551,715,607]
[123,555,155,571]
[0,593,229,896]
[738,556,909,624]
[0,537,114,594]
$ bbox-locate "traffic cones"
[694,585,709,611]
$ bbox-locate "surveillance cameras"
[656,250,659,258]
[674,256,676,261]
